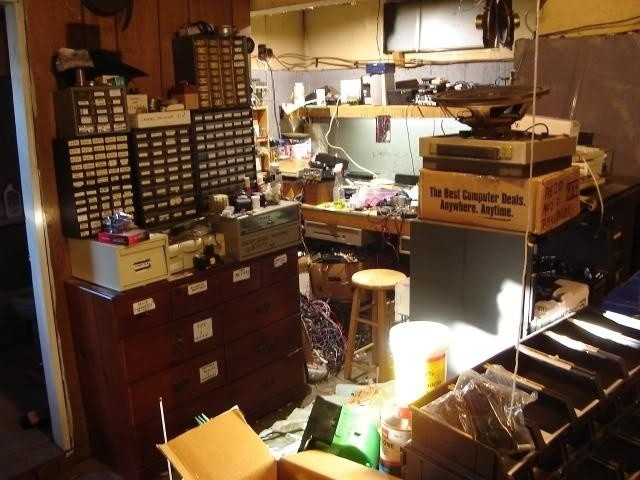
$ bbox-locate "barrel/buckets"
[378,419,414,474]
[388,319,451,406]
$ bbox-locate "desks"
[410,176,640,340]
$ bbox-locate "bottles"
[293,82,305,106]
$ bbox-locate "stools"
[344,269,407,383]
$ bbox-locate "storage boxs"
[156,406,405,480]
[418,165,580,235]
[308,260,373,303]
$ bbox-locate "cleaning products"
[330,163,345,208]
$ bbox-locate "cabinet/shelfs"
[63,242,311,480]
[279,104,507,236]
